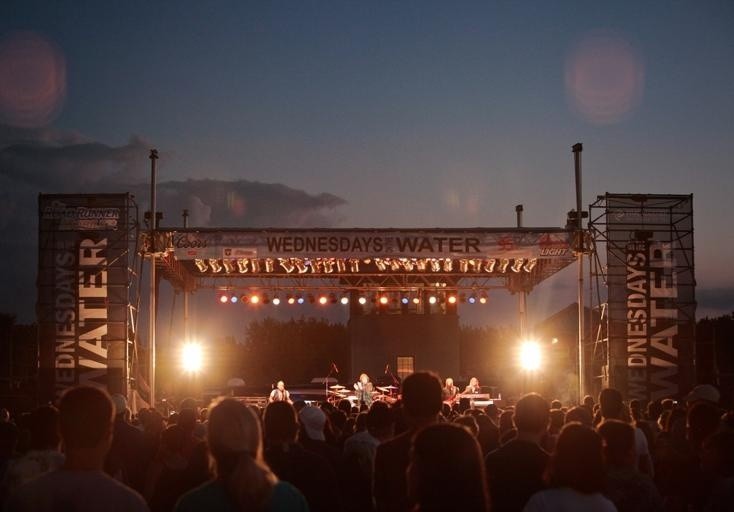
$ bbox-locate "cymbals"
[338,389,351,392]
[376,387,389,392]
[385,386,399,389]
[336,394,346,397]
[330,386,346,389]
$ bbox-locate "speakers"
[361,282,449,316]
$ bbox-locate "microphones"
[331,363,339,373]
[384,364,389,374]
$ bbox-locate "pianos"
[461,393,490,401]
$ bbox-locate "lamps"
[219,294,489,304]
[195,258,538,274]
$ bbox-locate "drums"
[371,395,391,409]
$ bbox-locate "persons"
[1,370,733,512]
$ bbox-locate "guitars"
[446,388,471,406]
